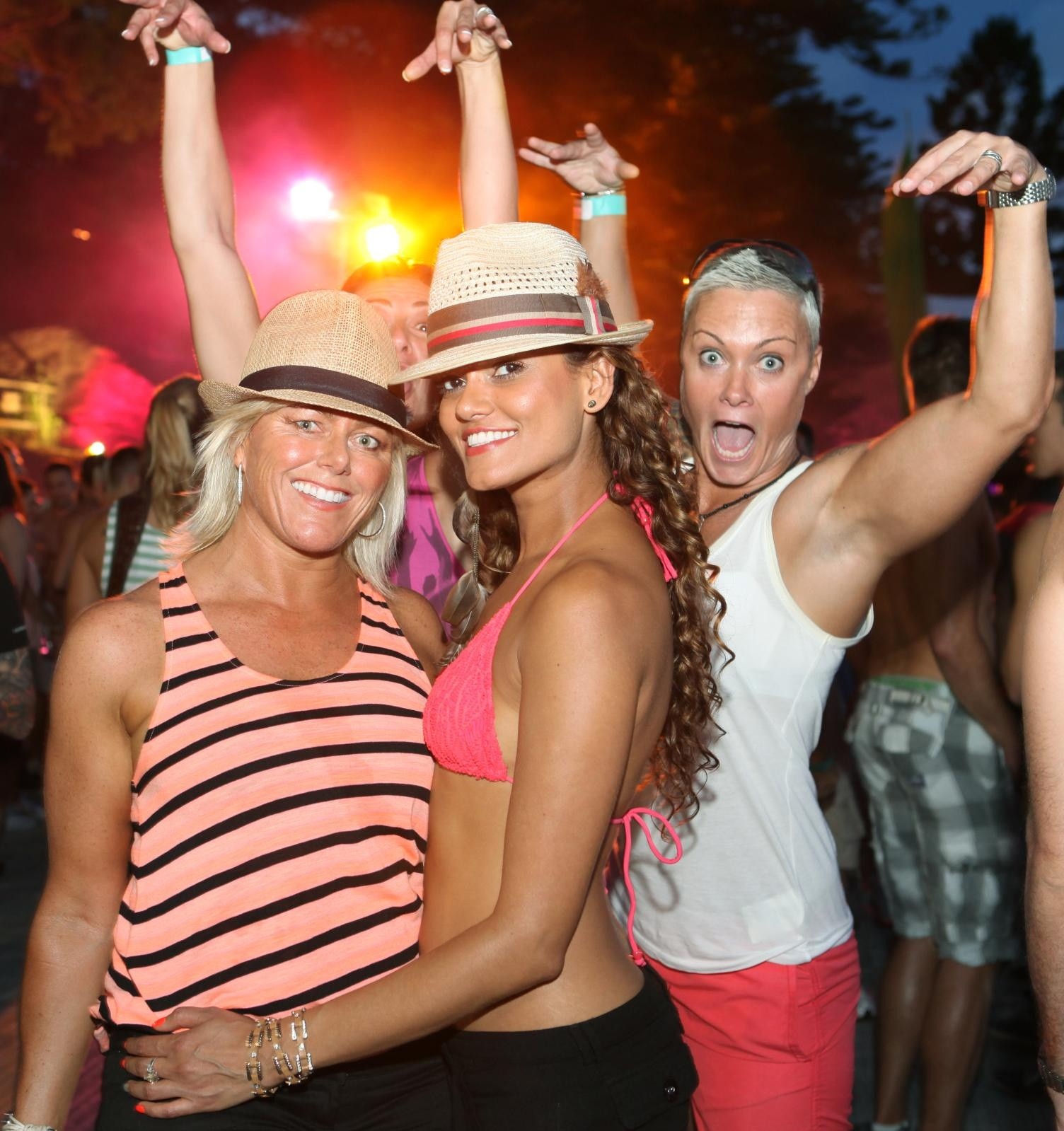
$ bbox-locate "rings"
[145,1057,160,1085]
[980,150,1002,171]
[476,6,493,17]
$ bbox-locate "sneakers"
[856,983,876,1020]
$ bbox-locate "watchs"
[978,165,1056,208]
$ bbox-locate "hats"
[198,290,442,456]
[384,221,654,387]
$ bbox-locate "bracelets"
[0,1112,57,1131]
[165,45,213,64]
[698,454,803,531]
[581,186,626,220]
[245,1007,314,1098]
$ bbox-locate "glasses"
[688,237,821,319]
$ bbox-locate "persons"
[0,0,1064,1131]
